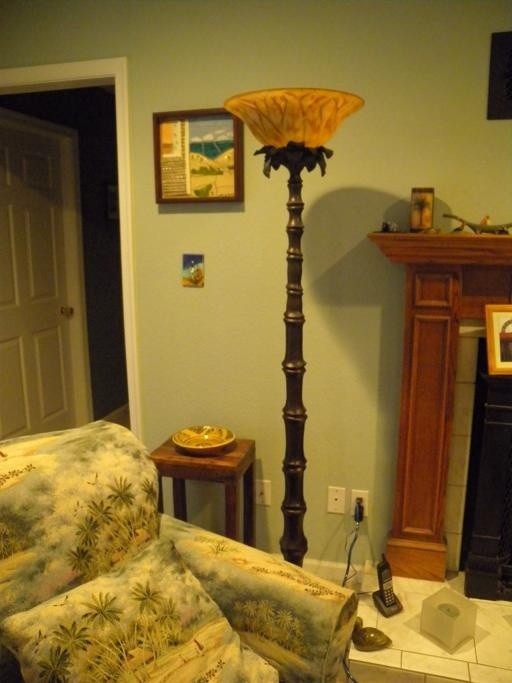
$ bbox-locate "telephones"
[373,552,402,617]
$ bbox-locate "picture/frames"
[484,302,512,376]
[151,107,246,205]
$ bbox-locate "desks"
[147,432,256,546]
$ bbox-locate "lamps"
[224,86,366,572]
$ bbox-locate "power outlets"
[351,490,369,518]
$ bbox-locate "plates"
[170,424,237,457]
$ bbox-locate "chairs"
[0,420,357,683]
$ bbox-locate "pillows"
[0,538,277,683]
